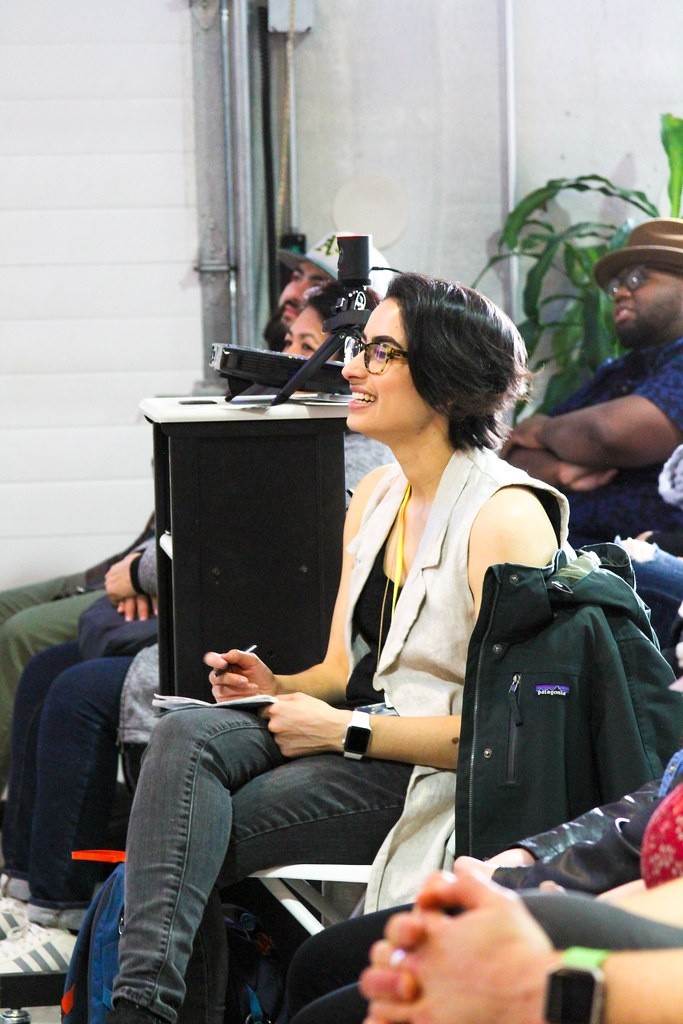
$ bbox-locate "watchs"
[544,946,610,1024]
[343,710,371,762]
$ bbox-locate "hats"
[594,217,682,287]
[277,232,391,301]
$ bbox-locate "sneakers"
[0,921,78,974]
[0,896,29,940]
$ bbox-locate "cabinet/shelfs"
[137,395,351,715]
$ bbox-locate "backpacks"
[61,860,229,1024]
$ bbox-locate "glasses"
[343,337,410,375]
[606,265,680,301]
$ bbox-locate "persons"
[98,273,568,1024]
[280,217,683,1024]
[0,233,400,976]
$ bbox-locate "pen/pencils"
[213,645,258,678]
[390,903,451,967]
